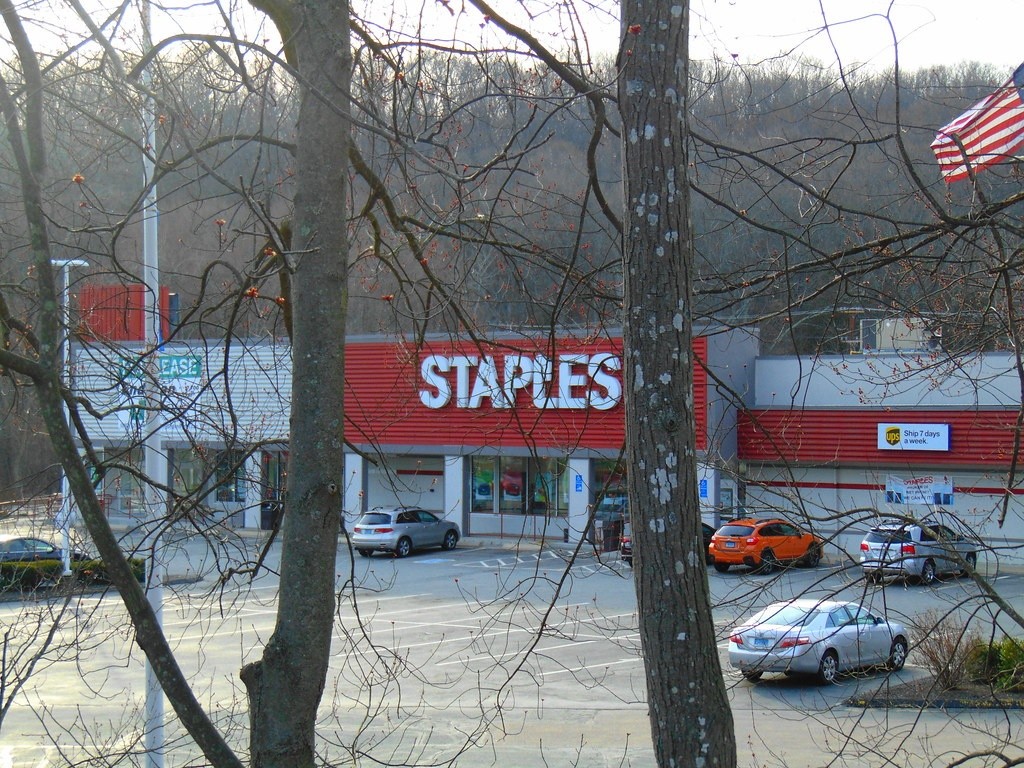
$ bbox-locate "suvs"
[859,516,977,586]
[708,516,824,575]
[352,503,462,558]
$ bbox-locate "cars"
[0,537,93,562]
[729,598,912,685]
[621,520,717,569]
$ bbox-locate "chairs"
[827,613,839,626]
[772,527,785,536]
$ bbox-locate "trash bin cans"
[602,520,625,552]
[261,501,281,530]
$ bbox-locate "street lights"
[49,258,91,578]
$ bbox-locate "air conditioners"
[859,317,943,354]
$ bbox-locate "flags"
[929,62,1024,186]
